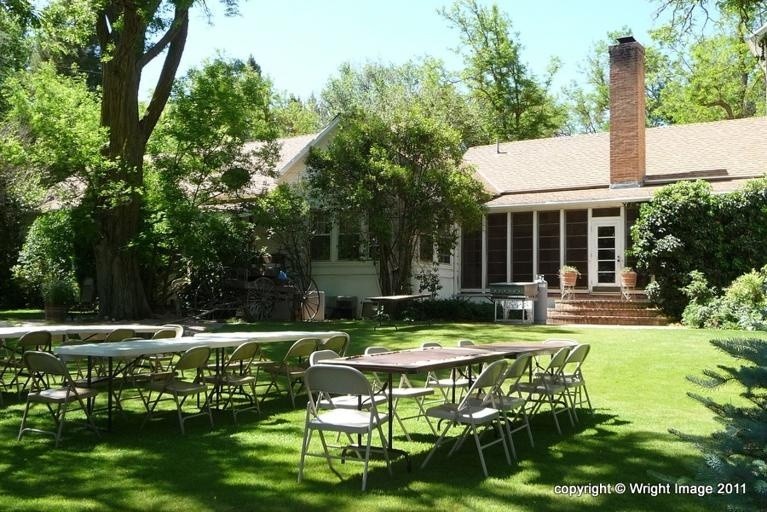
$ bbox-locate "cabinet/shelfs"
[561,284,575,300]
[621,284,634,303]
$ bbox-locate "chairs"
[0,323,593,491]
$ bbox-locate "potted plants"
[563,265,578,286]
[619,266,637,288]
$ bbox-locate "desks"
[365,294,433,332]
[490,295,535,324]
